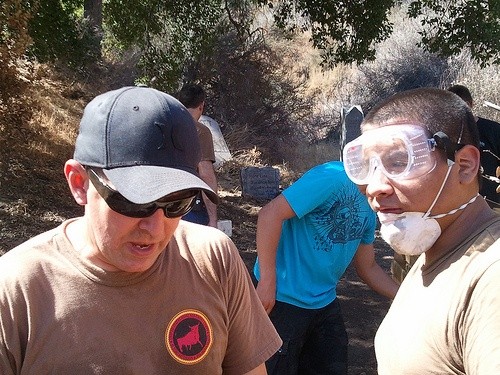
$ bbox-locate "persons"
[240,149,399,375]
[0,85,283,375]
[452,85,500,206]
[175,84,220,229]
[340,88,500,375]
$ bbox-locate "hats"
[73,84,220,207]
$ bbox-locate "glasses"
[342,120,468,185]
[84,164,201,219]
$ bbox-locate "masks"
[379,160,480,256]
